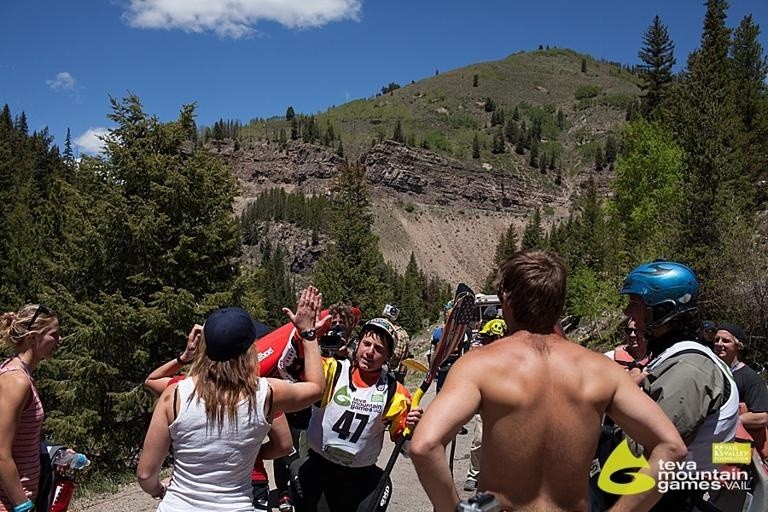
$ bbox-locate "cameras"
[382,304,400,322]
[321,325,345,357]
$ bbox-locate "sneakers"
[459,427,468,435]
[464,480,477,491]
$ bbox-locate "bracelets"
[175,352,193,365]
[13,500,33,510]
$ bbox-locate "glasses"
[625,327,640,336]
[27,304,49,331]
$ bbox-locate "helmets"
[364,318,398,351]
[619,262,699,328]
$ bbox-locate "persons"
[411,249,688,511]
[0,303,77,511]
[136,284,424,512]
[412,250,766,512]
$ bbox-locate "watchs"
[297,329,317,340]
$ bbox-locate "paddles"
[401,358,430,373]
[367,294,475,512]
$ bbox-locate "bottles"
[44,476,74,512]
[52,450,91,471]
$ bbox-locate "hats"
[716,323,747,343]
[204,308,268,361]
